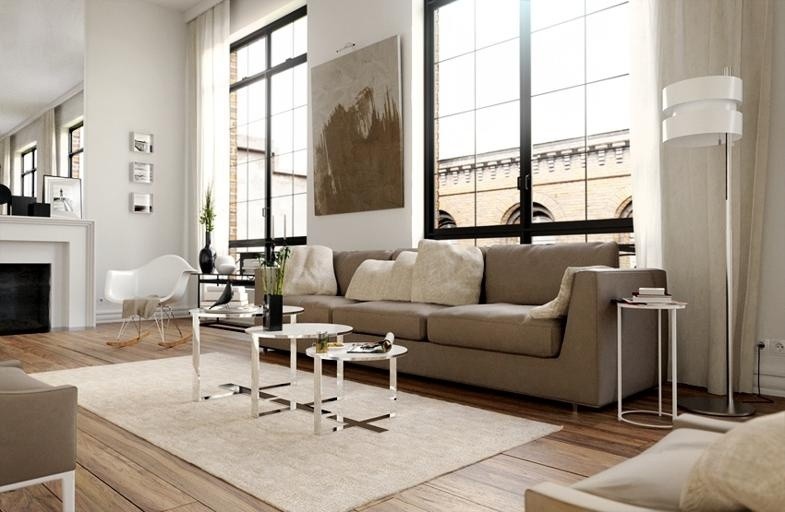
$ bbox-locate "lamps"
[658,71,757,421]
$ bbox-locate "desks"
[613,301,688,430]
[183,269,255,334]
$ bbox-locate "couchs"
[1,357,79,512]
[520,412,744,512]
[252,240,670,414]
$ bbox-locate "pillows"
[281,244,338,297]
[526,264,614,322]
[381,250,417,300]
[676,408,785,512]
[409,238,485,307]
[343,258,395,303]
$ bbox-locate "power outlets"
[769,338,785,359]
[754,338,769,357]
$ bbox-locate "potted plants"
[254,243,292,332]
[197,185,217,275]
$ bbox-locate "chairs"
[101,253,198,349]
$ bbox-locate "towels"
[120,294,166,321]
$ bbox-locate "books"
[632,287,673,303]
[347,332,394,353]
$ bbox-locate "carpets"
[27,349,566,512]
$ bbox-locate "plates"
[328,345,346,350]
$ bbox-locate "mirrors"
[0,0,88,217]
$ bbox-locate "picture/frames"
[42,174,84,220]
[128,130,155,215]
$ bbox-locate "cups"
[316,332,328,353]
